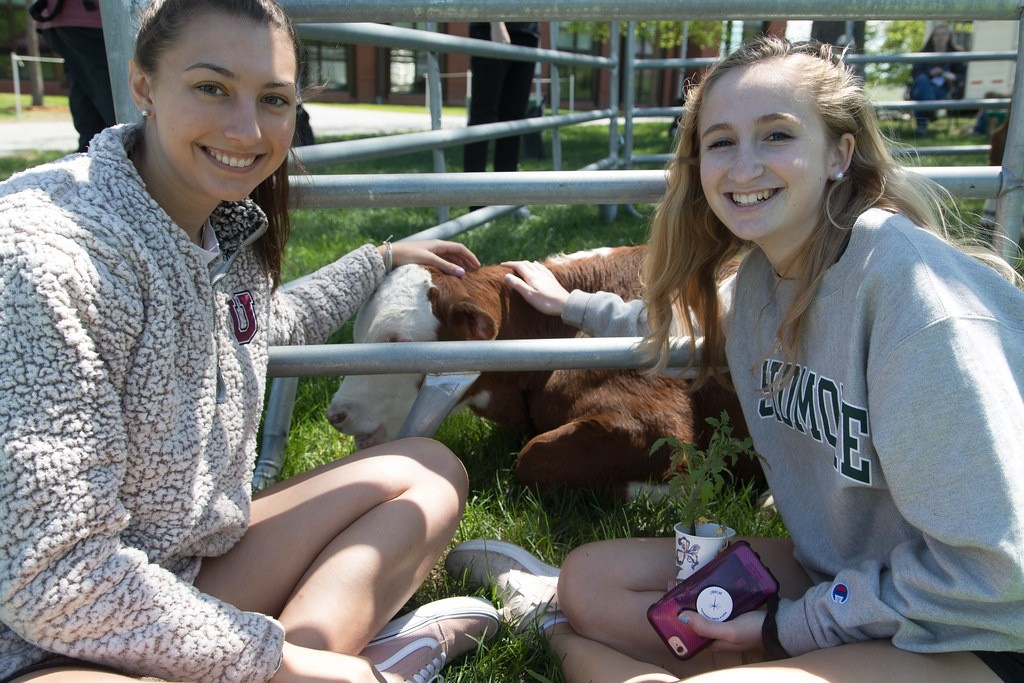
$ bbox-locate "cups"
[673,523,737,586]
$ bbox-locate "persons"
[906,25,968,135]
[445,34,1024,683]
[29,0,121,153]
[0,0,499,683]
[463,17,542,219]
[982,120,1023,234]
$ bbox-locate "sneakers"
[359,597,500,683]
[446,539,562,635]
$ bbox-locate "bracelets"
[383,235,394,273]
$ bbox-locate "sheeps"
[326,244,775,542]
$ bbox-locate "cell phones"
[647,539,780,661]
